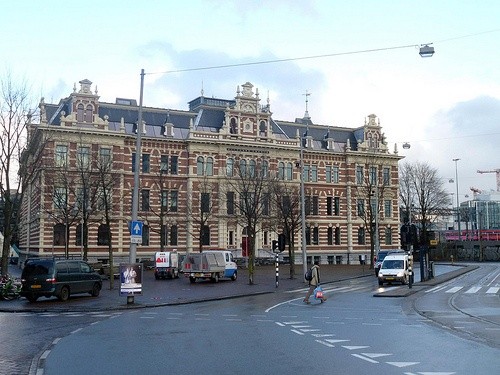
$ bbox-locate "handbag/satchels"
[316,291,323,298]
[313,285,324,300]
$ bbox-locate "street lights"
[453,158,461,242]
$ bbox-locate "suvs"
[373,250,412,285]
[21,257,103,302]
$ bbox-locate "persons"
[123,267,137,283]
[303,261,328,303]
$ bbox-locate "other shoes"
[321,299,327,303]
[303,299,311,304]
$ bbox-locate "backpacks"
[305,267,315,281]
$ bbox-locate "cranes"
[477,169,500,192]
[470,186,480,199]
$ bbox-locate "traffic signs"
[131,220,143,243]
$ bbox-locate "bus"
[443,229,500,241]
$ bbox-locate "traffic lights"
[272,241,278,251]
[278,234,285,251]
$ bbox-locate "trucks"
[183,251,237,283]
